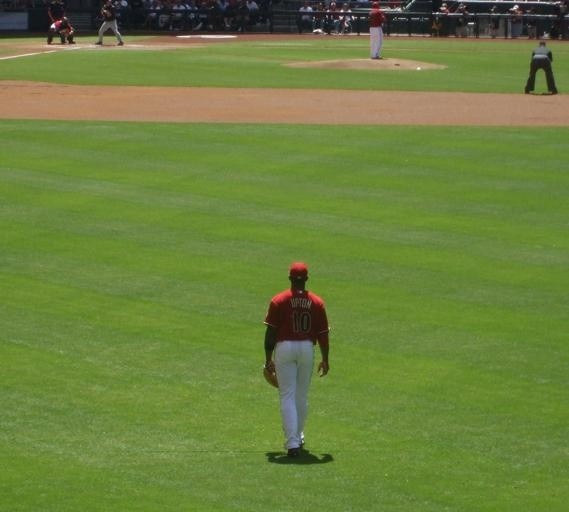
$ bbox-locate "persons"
[262,261,331,459]
[367,1,385,59]
[524,41,557,96]
[428,1,569,41]
[298,0,355,35]
[46,0,259,46]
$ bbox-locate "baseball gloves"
[263,361,278,387]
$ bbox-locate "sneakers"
[62,42,76,44]
[372,57,382,59]
[95,42,102,46]
[287,439,304,456]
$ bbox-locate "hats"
[290,263,308,277]
[372,2,379,8]
